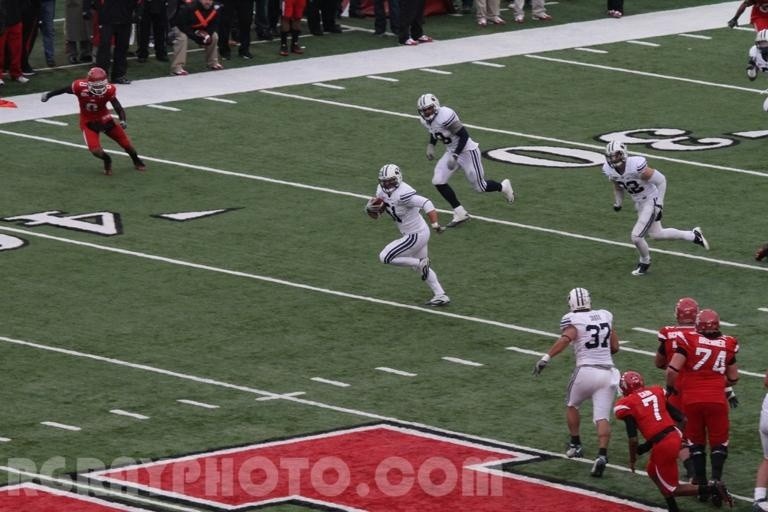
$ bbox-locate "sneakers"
[103,154,112,175]
[238,49,253,60]
[631,262,651,276]
[17,75,29,83]
[110,74,131,85]
[532,12,552,21]
[445,211,470,228]
[132,157,146,171]
[399,38,418,45]
[502,178,515,206]
[590,456,609,477]
[279,44,289,56]
[425,294,451,307]
[566,442,583,458]
[290,42,305,54]
[752,498,768,512]
[419,256,432,281]
[210,63,224,71]
[488,15,504,24]
[169,68,189,76]
[477,18,487,26]
[692,225,710,250]
[21,67,36,75]
[714,480,735,508]
[418,34,432,42]
[514,15,524,23]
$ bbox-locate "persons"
[727,0,768,32]
[754,243,768,261]
[371,0,399,35]
[655,297,740,484]
[665,309,740,507]
[606,0,624,19]
[602,141,710,275]
[752,371,768,512]
[508,0,552,22]
[531,287,621,477]
[475,0,506,26]
[399,0,432,45]
[416,93,514,228]
[363,163,450,306]
[745,29,768,112]
[40,68,146,176]
[613,371,734,512]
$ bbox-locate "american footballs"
[371,198,386,215]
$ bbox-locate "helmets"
[377,163,402,194]
[619,370,645,396]
[566,287,592,312]
[695,308,720,333]
[416,93,440,121]
[87,66,109,96]
[674,297,700,325]
[604,139,628,168]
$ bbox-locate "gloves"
[431,221,447,234]
[724,386,739,409]
[613,203,622,211]
[365,198,382,213]
[119,119,127,129]
[40,91,48,103]
[425,144,437,161]
[531,353,551,375]
[446,153,458,170]
[654,202,664,221]
